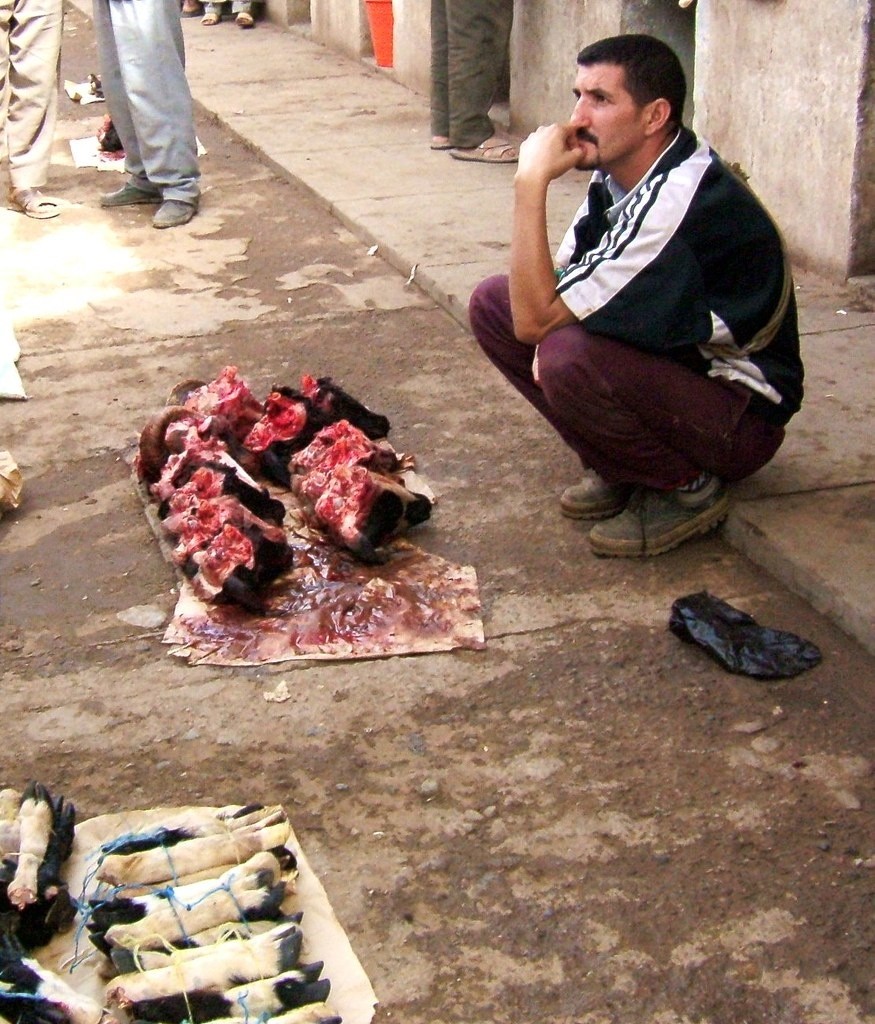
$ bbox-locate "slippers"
[430,138,452,149]
[180,6,204,18]
[450,138,520,162]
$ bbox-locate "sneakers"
[560,470,638,519]
[154,200,196,227]
[587,473,735,559]
[98,181,163,206]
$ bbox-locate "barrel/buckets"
[365,0,394,68]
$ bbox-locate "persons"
[431,0,520,163]
[468,34,804,559]
[0,0,65,219]
[91,0,200,228]
[181,0,256,25]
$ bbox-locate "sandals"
[6,186,61,219]
[201,13,222,25]
[235,12,253,25]
